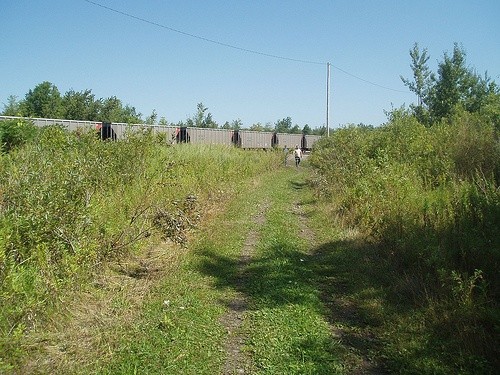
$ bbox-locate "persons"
[294,144,303,166]
[283,145,289,163]
